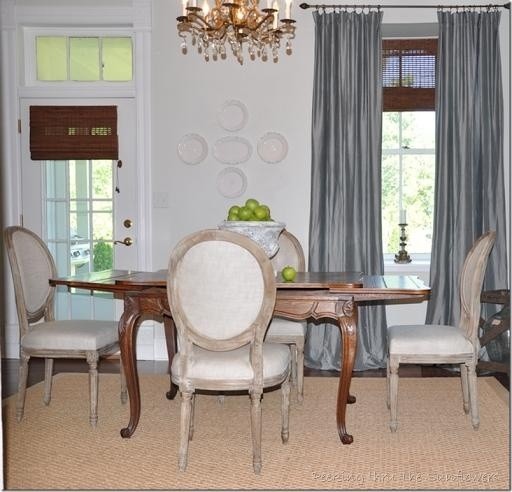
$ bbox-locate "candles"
[400,209,407,223]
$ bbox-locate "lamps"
[177,0,296,66]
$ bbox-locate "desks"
[45,269,431,445]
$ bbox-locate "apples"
[228,199,272,221]
[282,265,296,281]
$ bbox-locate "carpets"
[2,372,511,491]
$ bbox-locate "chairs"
[3,226,127,426]
[385,229,497,432]
[166,228,291,474]
[264,230,306,403]
